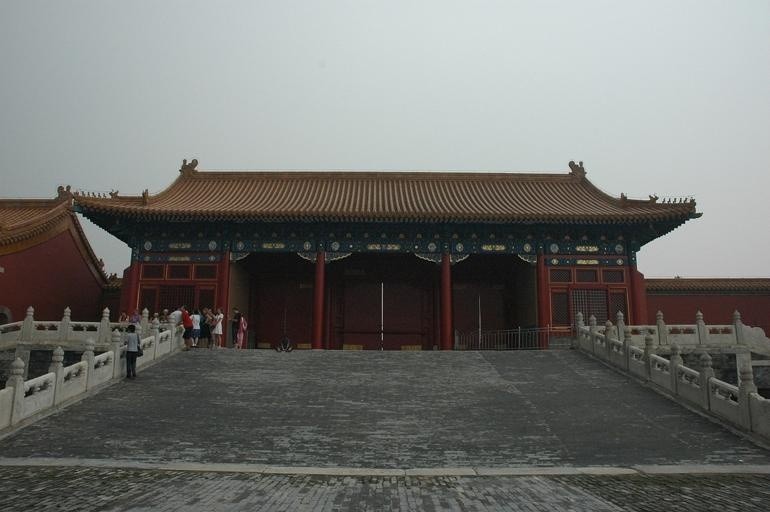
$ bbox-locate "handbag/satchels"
[137,345,144,357]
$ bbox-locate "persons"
[117,303,248,379]
